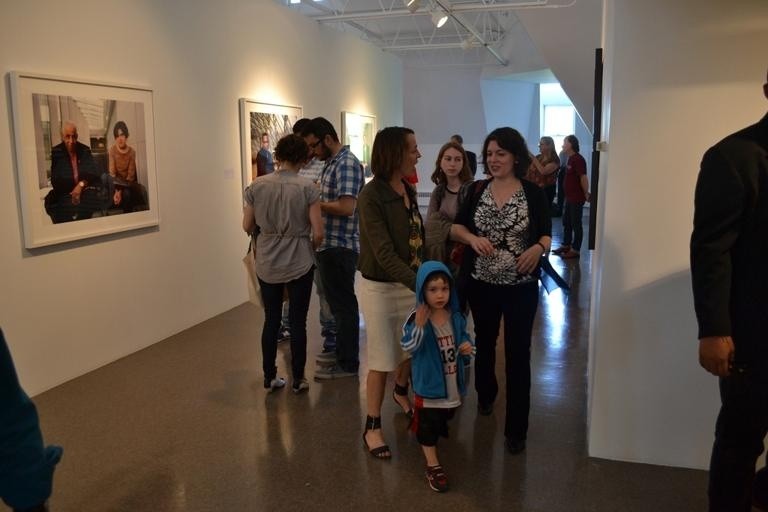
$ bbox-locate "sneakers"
[423,463,450,493]
[322,335,336,349]
[263,377,285,392]
[276,328,292,343]
[314,364,358,378]
[292,378,312,393]
[316,352,336,362]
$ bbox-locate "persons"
[687,76,767,512]
[526,135,562,217]
[255,133,275,177]
[557,150,568,216]
[99,120,150,215]
[448,125,554,456]
[400,166,418,207]
[419,142,477,328]
[549,135,593,261]
[447,134,476,175]
[299,117,365,382]
[41,122,100,225]
[351,125,427,461]
[276,118,339,365]
[399,260,478,493]
[1,329,63,510]
[240,134,325,395]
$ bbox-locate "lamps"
[401,0,454,31]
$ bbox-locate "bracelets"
[536,242,546,251]
[78,181,84,187]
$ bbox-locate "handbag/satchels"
[243,234,287,306]
[446,242,470,265]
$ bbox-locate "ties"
[70,151,78,184]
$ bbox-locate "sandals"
[361,415,392,462]
[392,382,415,419]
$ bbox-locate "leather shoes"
[561,249,579,258]
[479,398,492,415]
[503,436,526,456]
[552,248,569,253]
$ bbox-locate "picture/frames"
[341,112,378,184]
[240,99,303,207]
[8,70,158,251]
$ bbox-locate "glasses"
[308,139,323,148]
